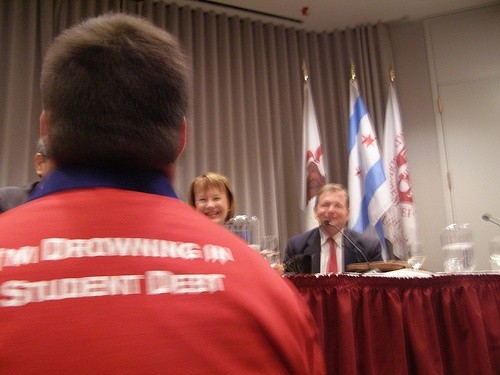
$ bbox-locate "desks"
[282,272,500,375]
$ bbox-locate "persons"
[0,138,49,213]
[189,172,236,229]
[0,11,324,375]
[284,184,383,275]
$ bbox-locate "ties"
[327,236,338,274]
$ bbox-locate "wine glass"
[408,255,425,277]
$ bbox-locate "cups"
[487,236,500,273]
[259,236,279,268]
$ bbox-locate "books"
[346,261,409,273]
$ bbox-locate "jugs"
[224,216,261,253]
[440,222,473,273]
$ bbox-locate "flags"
[298,82,329,234]
[381,83,417,262]
[349,80,394,262]
